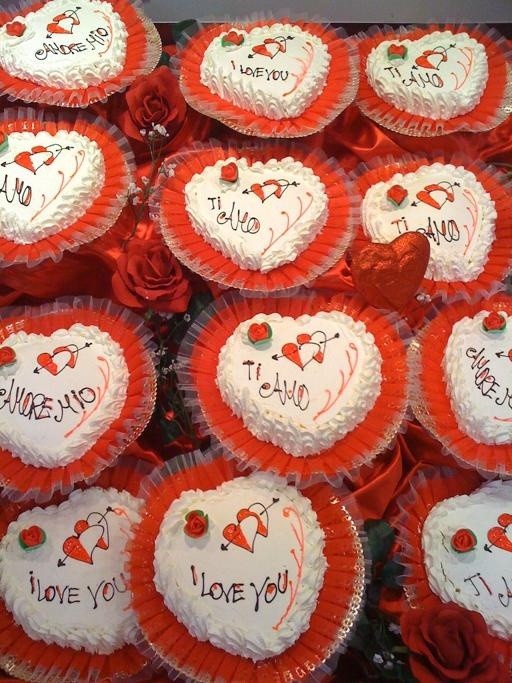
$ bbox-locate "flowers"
[115,69,195,160]
[110,240,195,314]
[400,595,492,683]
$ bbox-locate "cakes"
[0,0,512,662]
